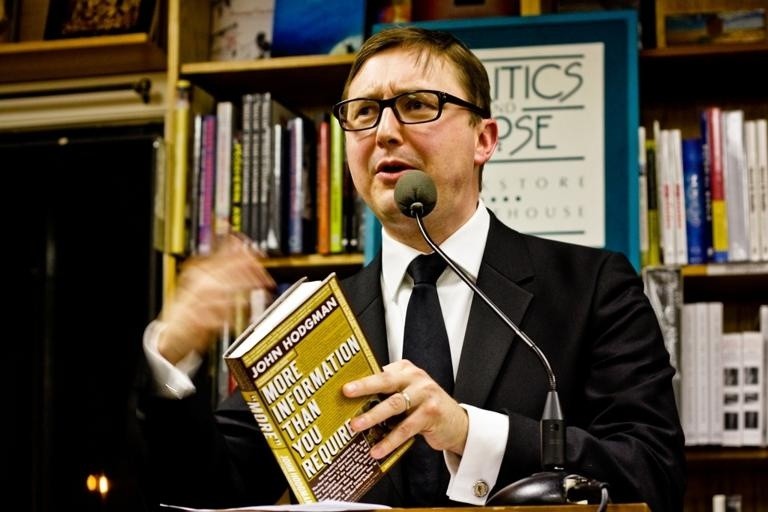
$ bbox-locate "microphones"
[394,170,612,507]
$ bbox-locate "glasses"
[330,87,490,132]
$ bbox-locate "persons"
[138,25,689,512]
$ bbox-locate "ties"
[399,251,454,508]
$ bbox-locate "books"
[220,272,416,504]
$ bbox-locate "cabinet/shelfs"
[161,0,767,461]
